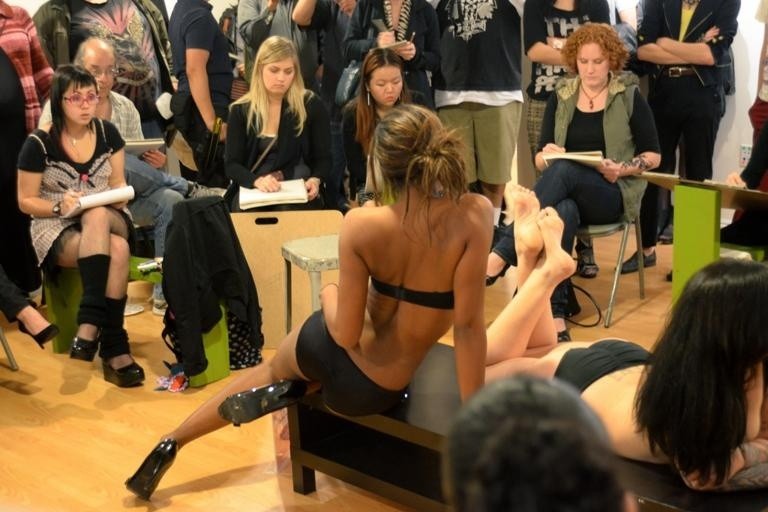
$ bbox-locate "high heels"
[575,237,599,277]
[124,439,180,501]
[18,312,59,350]
[216,377,307,426]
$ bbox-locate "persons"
[486,181,767,491]
[125,105,493,502]
[439,371,637,512]
[0,0,768,389]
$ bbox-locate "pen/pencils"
[409,31,416,41]
[58,181,70,191]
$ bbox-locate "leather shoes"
[615,248,657,274]
[70,323,100,361]
[485,256,512,286]
[554,315,571,341]
[101,354,146,388]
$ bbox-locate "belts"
[658,66,697,79]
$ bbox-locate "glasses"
[90,65,117,76]
[63,91,97,104]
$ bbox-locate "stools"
[281,230,340,336]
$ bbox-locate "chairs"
[572,212,646,330]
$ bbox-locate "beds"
[287,342,768,510]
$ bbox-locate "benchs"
[39,252,231,390]
